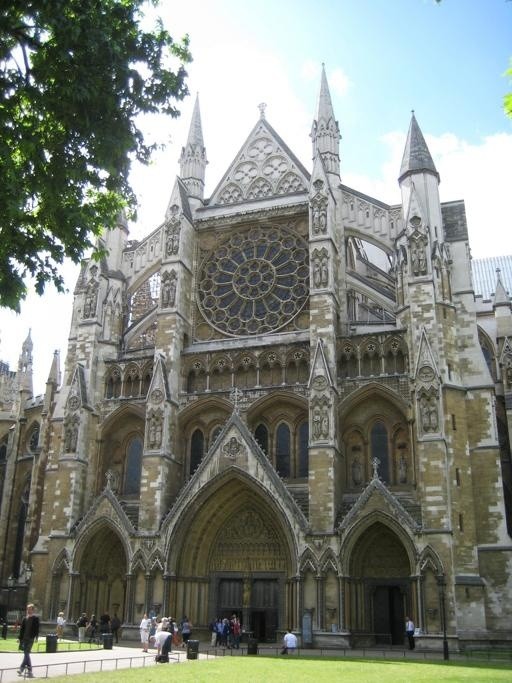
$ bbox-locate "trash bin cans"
[102,633,113,648]
[248,638,257,654]
[187,640,200,659]
[46,634,57,653]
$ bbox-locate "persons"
[56,612,66,639]
[149,631,173,663]
[403,614,416,650]
[0,617,9,641]
[75,611,121,644]
[138,613,194,652]
[279,629,298,655]
[18,602,41,676]
[210,615,242,651]
